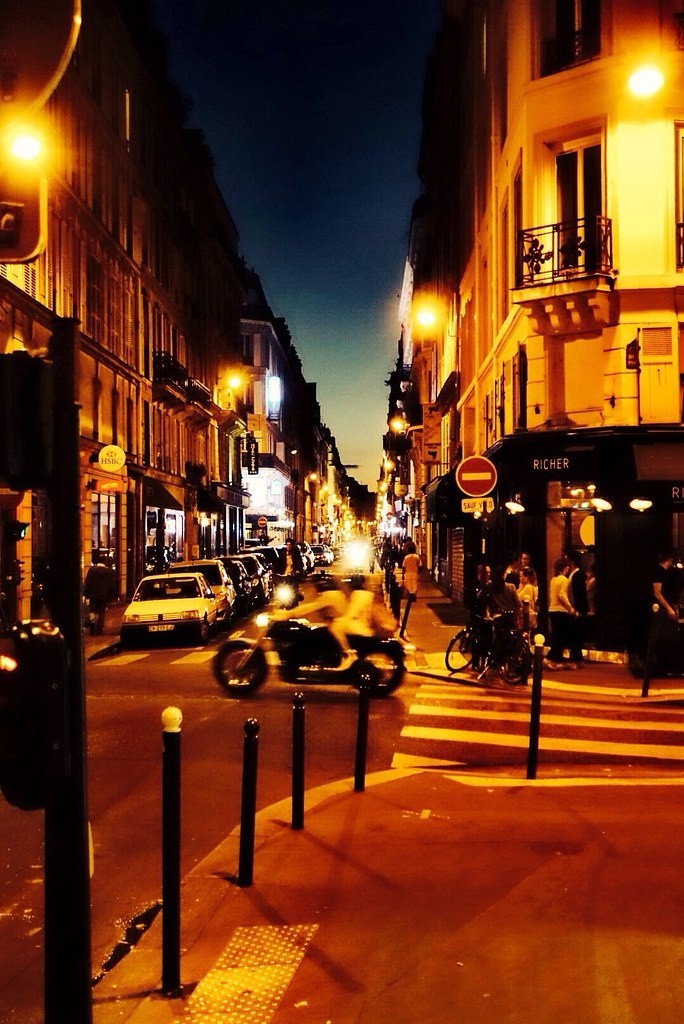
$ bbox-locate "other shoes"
[543,657,559,670]
[338,649,358,671]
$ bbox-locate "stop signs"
[455,455,498,498]
[257,517,268,527]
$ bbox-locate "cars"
[168,558,238,629]
[118,572,217,644]
[210,538,334,613]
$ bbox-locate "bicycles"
[444,600,532,685]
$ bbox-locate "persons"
[83,554,120,637]
[642,552,677,678]
[469,554,539,683]
[571,551,605,662]
[543,557,580,670]
[276,537,304,610]
[402,543,422,602]
[264,574,381,670]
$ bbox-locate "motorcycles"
[211,582,409,697]
